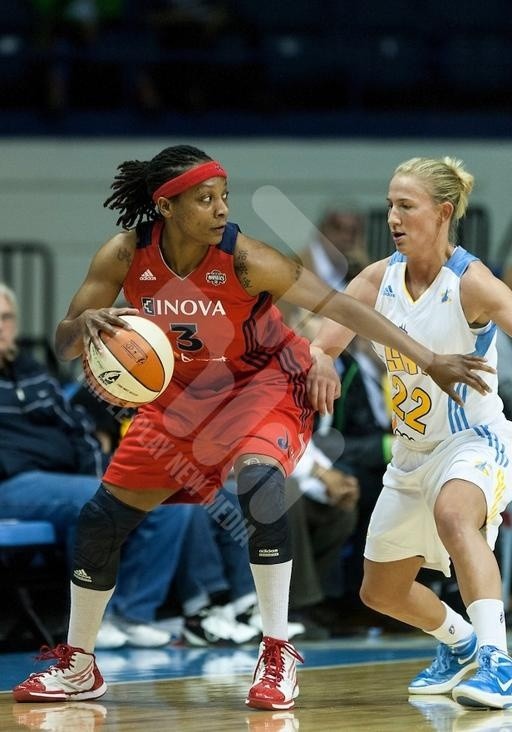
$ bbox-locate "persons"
[9,141,500,717]
[282,439,391,639]
[71,389,305,650]
[12,645,511,731]
[0,279,194,655]
[324,331,472,624]
[295,202,374,296]
[303,150,512,714]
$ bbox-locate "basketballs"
[82,316,175,409]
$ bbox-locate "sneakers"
[407,631,480,695]
[451,645,512,710]
[245,636,304,711]
[12,643,109,703]
[94,603,306,650]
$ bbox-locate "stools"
[2,514,58,651]
